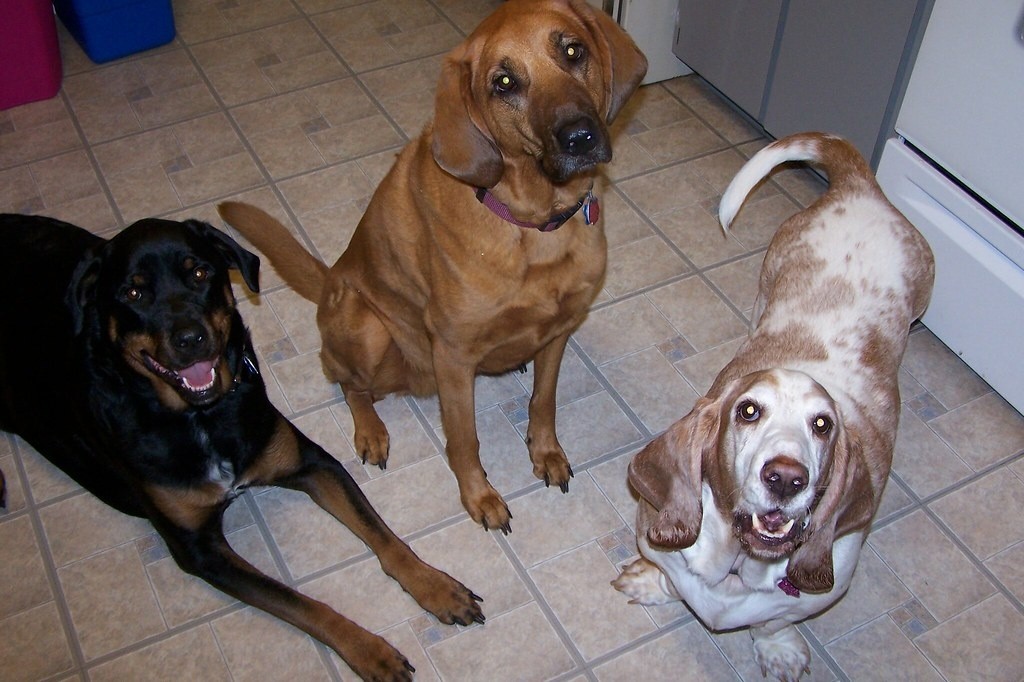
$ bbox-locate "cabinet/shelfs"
[672,0,1024,418]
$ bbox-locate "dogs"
[218,1,649,537]
[610,130,936,682]
[0,214,486,682]
